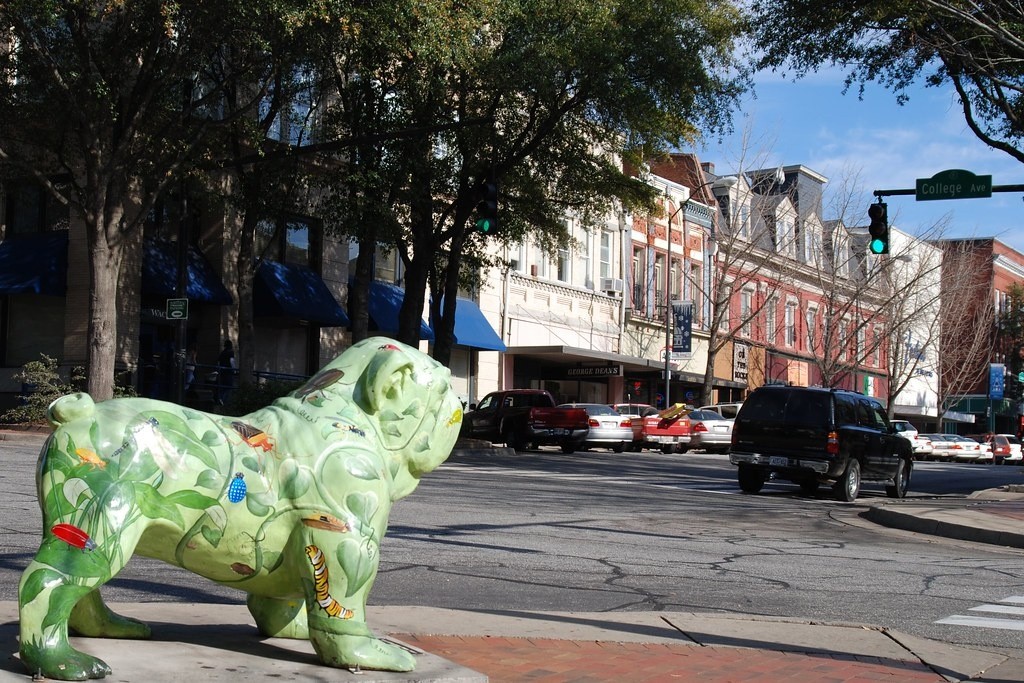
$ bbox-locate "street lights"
[665,177,740,409]
[854,255,914,392]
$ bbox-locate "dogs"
[17,336,465,681]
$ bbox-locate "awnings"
[138,236,235,305]
[348,276,436,340]
[0,232,67,298]
[429,293,506,351]
[254,257,350,328]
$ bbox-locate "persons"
[183,342,199,393]
[216,339,239,405]
[986,431,996,465]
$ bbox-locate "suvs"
[729,383,915,502]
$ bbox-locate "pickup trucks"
[607,404,691,454]
[458,389,591,455]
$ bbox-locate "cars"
[878,419,919,449]
[557,404,636,453]
[910,432,1024,466]
[685,404,735,455]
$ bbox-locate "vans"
[698,403,743,422]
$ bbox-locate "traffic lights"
[476,172,500,236]
[867,203,889,254]
